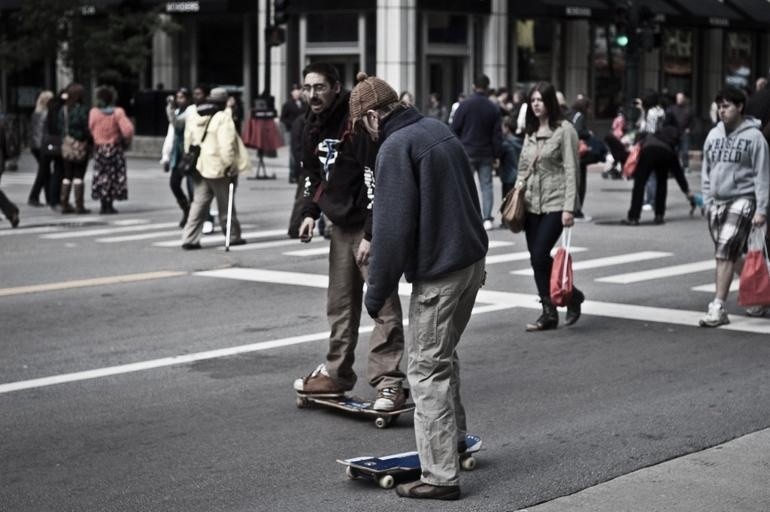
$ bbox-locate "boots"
[564,285,584,326]
[525,299,558,331]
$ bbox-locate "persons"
[602,92,697,225]
[293,61,411,412]
[181,88,247,251]
[727,76,770,141]
[489,83,528,223]
[27,79,134,216]
[349,72,490,501]
[513,80,584,330]
[555,91,594,224]
[230,81,306,183]
[698,85,770,327]
[165,85,216,235]
[447,71,506,232]
[401,93,465,124]
[1,129,19,228]
[159,86,190,228]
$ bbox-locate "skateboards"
[336,433,488,489]
[295,390,417,429]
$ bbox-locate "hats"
[349,71,398,118]
[210,87,228,102]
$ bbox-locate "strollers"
[603,132,633,180]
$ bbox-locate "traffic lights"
[265,25,286,48]
[610,32,630,72]
[275,1,291,24]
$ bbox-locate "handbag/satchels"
[737,250,770,306]
[179,145,203,182]
[502,188,526,233]
[61,135,88,162]
[580,136,607,163]
[42,135,60,156]
[549,249,573,306]
[623,141,641,177]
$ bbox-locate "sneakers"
[294,363,344,393]
[745,306,769,316]
[396,479,461,501]
[574,214,591,222]
[483,220,493,230]
[699,302,729,327]
[9,207,19,227]
[621,216,665,225]
[179,210,246,249]
[28,181,118,214]
[373,387,405,412]
